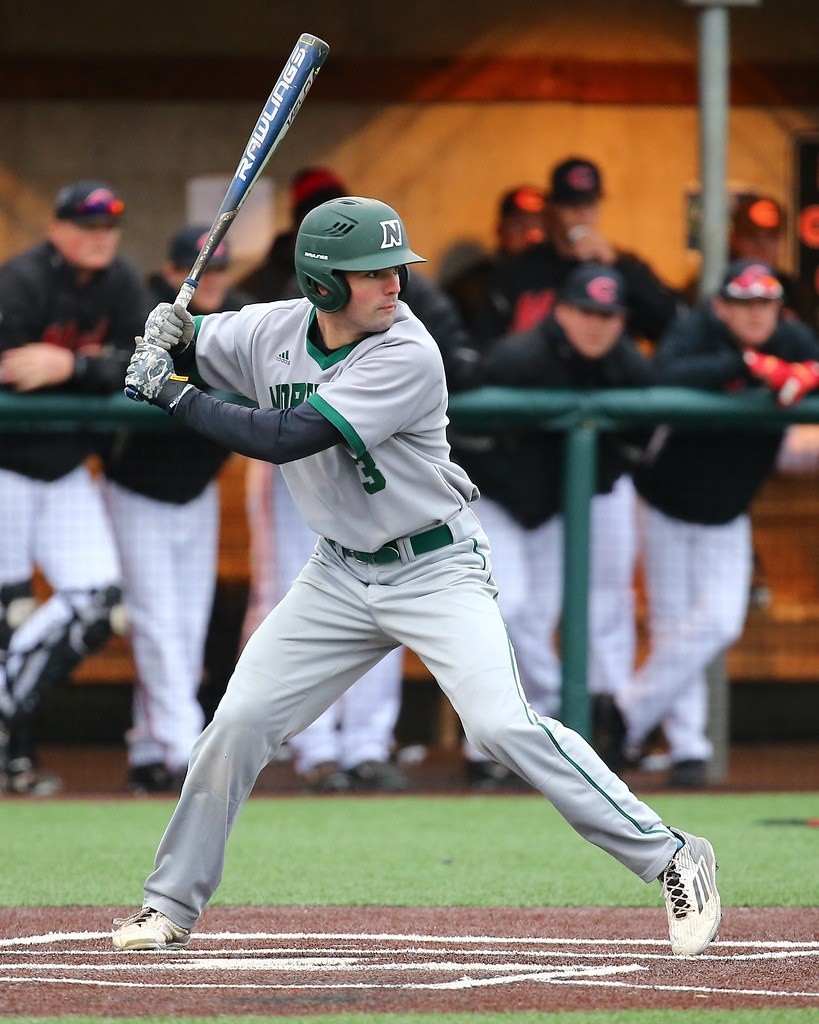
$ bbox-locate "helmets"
[293,196,429,314]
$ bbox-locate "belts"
[327,524,454,565]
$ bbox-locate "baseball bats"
[123,32,330,402]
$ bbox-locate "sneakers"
[657,825,722,956]
[110,905,192,951]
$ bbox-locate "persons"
[453,263,656,779]
[115,193,715,958]
[590,263,819,795]
[0,179,155,795]
[461,158,819,769]
[101,165,493,798]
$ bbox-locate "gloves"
[778,358,819,408]
[142,301,196,359]
[742,346,792,390]
[124,335,189,416]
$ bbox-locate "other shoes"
[305,759,410,796]
[464,759,515,783]
[608,756,643,781]
[126,763,184,795]
[0,757,70,799]
[667,760,710,789]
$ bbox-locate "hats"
[499,185,553,220]
[51,178,126,227]
[556,263,631,318]
[721,256,785,302]
[544,158,602,207]
[166,222,230,270]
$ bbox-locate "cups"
[566,223,596,261]
